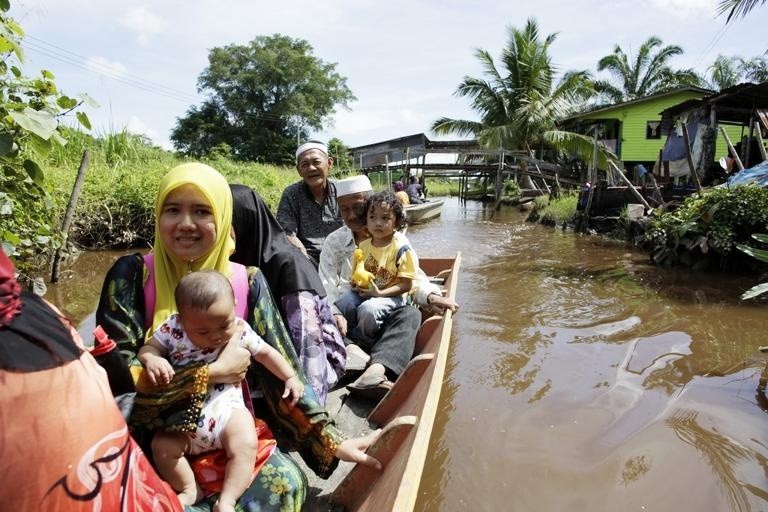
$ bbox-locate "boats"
[397,198,446,226]
[298,244,465,510]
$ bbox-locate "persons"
[93,162,384,512]
[393,174,427,204]
[331,190,419,351]
[0,241,189,510]
[274,138,349,274]
[132,266,308,511]
[317,175,460,400]
[227,183,347,418]
[700,151,739,189]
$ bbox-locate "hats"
[295,140,329,161]
[334,175,373,198]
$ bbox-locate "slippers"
[345,351,366,370]
[346,375,390,398]
